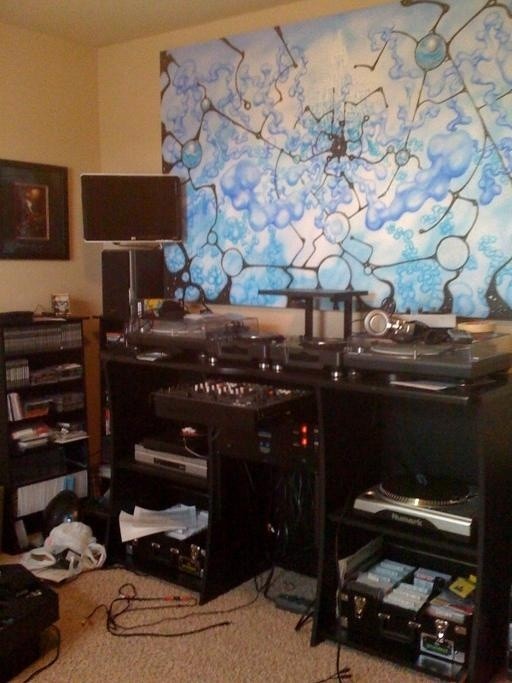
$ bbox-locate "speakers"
[101,248,164,317]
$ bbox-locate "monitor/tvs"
[79,173,183,243]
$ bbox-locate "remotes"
[446,328,473,342]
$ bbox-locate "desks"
[99,337,511,682]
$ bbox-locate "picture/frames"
[1,156,72,261]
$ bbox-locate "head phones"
[363,309,426,343]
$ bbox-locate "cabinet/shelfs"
[2,314,103,555]
